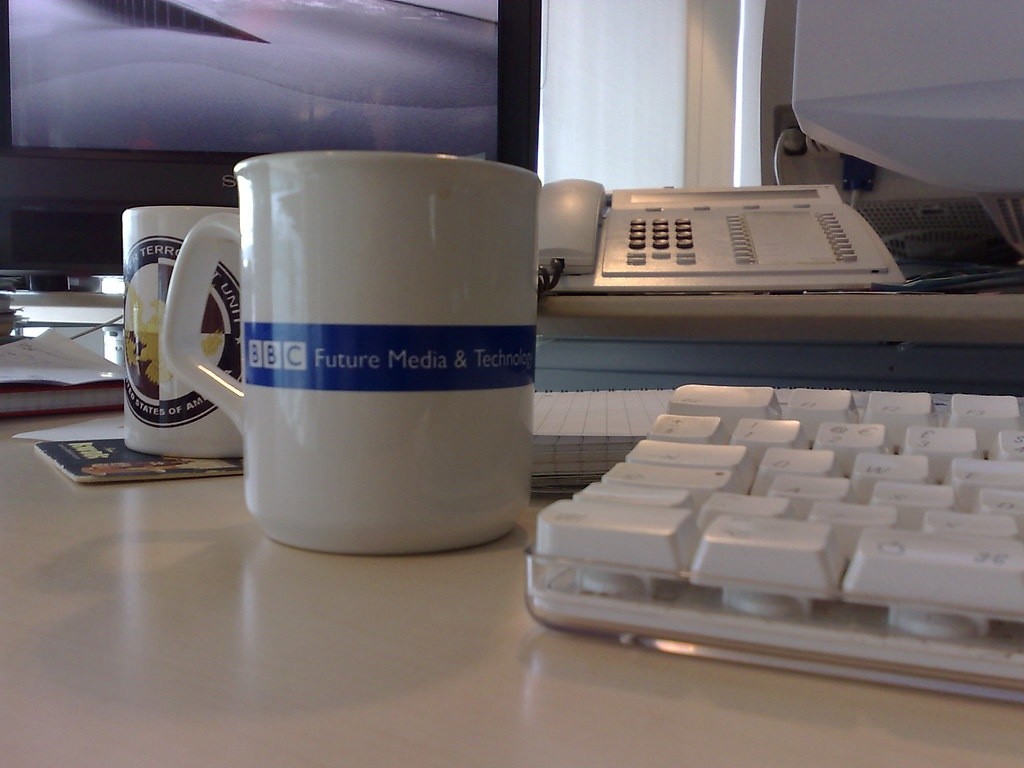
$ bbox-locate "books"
[531,387,677,497]
[0,383,125,416]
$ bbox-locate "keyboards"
[523,382,1023,705]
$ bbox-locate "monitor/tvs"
[0,0,542,275]
[735,0,1024,288]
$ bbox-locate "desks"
[0,407,1024,768]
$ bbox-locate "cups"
[117,203,248,463]
[156,148,545,556]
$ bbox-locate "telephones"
[533,176,908,297]
